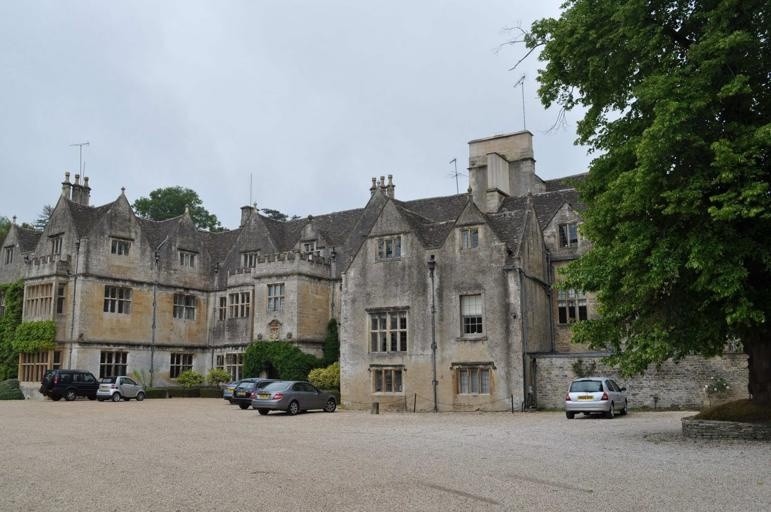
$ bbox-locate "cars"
[564,376,627,419]
[221,378,242,405]
[93,375,145,403]
[250,380,336,415]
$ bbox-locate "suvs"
[38,366,102,402]
[229,378,282,411]
